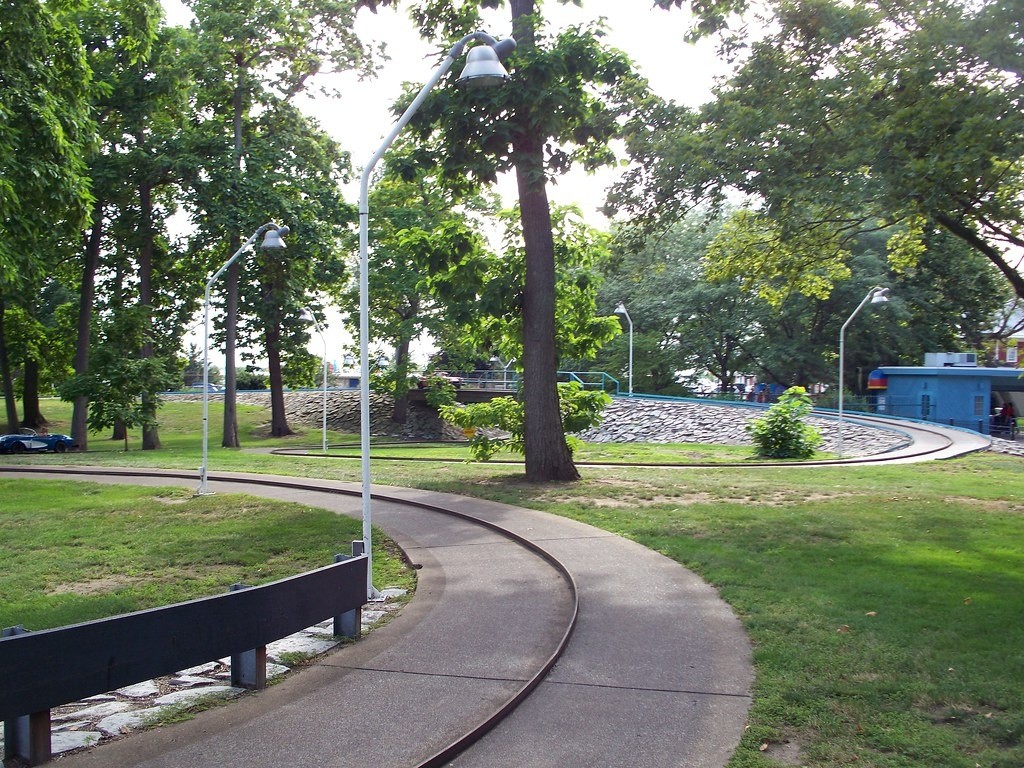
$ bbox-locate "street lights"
[195,221,288,499]
[356,31,519,599]
[489,355,517,390]
[297,305,330,453]
[614,302,635,395]
[839,285,892,460]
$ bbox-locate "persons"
[1000,403,1020,434]
[748,388,764,402]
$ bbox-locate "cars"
[705,385,743,401]
[676,379,717,398]
[416,372,465,391]
[729,382,746,394]
[192,381,226,393]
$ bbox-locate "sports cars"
[0,427,76,455]
